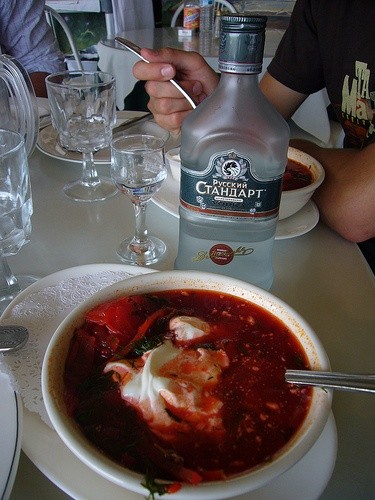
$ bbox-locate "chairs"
[44,4,85,72]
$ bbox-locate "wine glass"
[45,70,122,202]
[0,127,42,313]
[108,134,177,267]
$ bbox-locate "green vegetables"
[73,293,250,498]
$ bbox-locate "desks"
[7,109,374,499]
[94,26,289,112]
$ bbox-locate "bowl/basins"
[38,269,336,499]
[165,145,331,220]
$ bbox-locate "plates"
[0,262,339,500]
[177,158,186,161]
[151,165,320,241]
[38,109,171,164]
[0,367,27,496]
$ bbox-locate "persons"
[0,0,66,98]
[133,0,375,272]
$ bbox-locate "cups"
[0,53,37,159]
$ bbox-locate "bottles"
[215,10,222,33]
[172,13,290,292]
[200,0,215,33]
